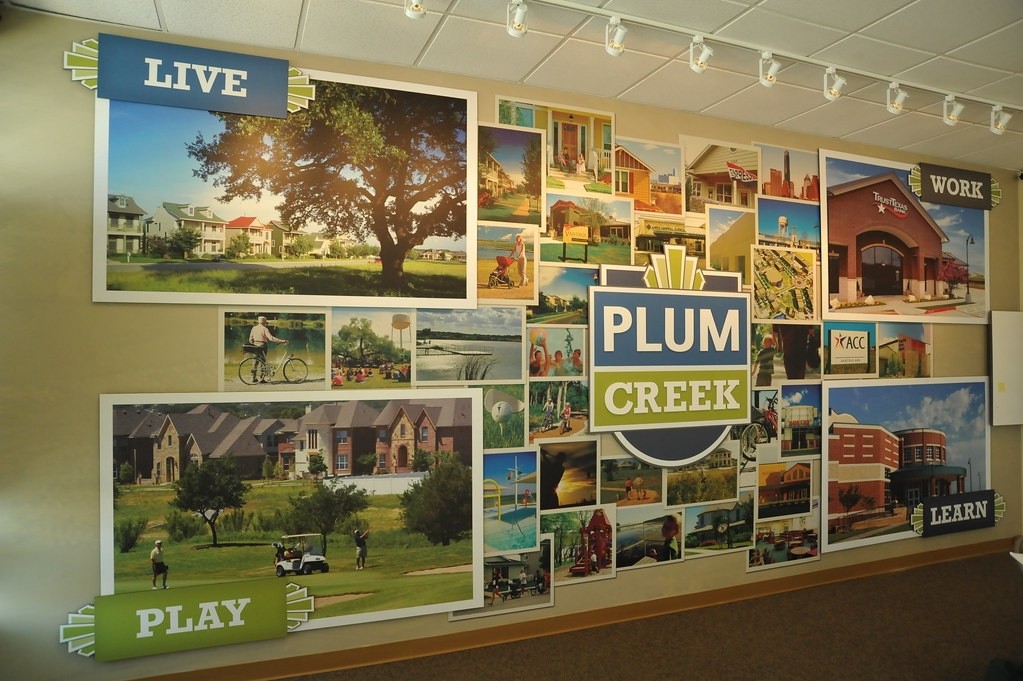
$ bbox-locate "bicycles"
[558,413,571,435]
[740,390,779,461]
[530,579,545,596]
[541,410,554,433]
[237,340,309,385]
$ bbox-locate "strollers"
[501,577,523,600]
[488,255,515,289]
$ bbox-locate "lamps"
[823,67,848,100]
[605,17,629,56]
[942,95,965,126]
[758,52,782,87]
[689,36,714,72]
[988,105,1012,135]
[885,82,908,114]
[404,0,427,18]
[505,0,529,38]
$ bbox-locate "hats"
[155,540,162,545]
[355,530,359,534]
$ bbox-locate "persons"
[331,361,410,386]
[772,323,815,380]
[528,338,584,377]
[651,512,682,563]
[542,398,555,429]
[633,474,644,500]
[558,146,573,173]
[577,153,586,174]
[751,334,779,387]
[249,315,289,384]
[589,550,600,573]
[353,530,369,569]
[535,569,546,594]
[151,540,171,590]
[625,477,633,500]
[519,570,530,598]
[508,232,529,287]
[487,567,507,605]
[561,402,571,432]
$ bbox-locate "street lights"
[507,468,524,511]
[963,234,976,304]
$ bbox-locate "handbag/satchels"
[806,334,821,369]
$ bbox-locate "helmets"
[548,398,553,402]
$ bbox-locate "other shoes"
[162,585,170,588]
[637,498,640,500]
[261,380,268,383]
[525,278,528,286]
[356,565,359,569]
[517,284,522,288]
[627,498,630,500]
[359,567,363,570]
[253,378,258,382]
[488,603,493,606]
[502,596,504,602]
[153,586,157,589]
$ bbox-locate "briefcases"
[242,344,256,353]
